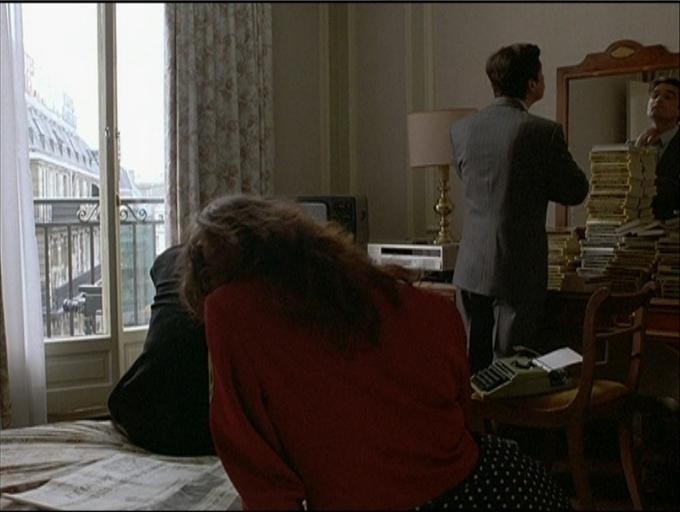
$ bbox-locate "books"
[542,142,680,300]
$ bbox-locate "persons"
[628,75,678,218]
[177,196,588,511]
[109,241,218,458]
[450,42,591,373]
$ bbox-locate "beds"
[0,420,244,512]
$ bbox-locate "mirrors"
[555,40,680,235]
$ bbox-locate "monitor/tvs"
[284,195,369,256]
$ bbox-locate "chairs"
[472,281,659,512]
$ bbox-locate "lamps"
[406,108,479,245]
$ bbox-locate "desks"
[414,279,680,343]
